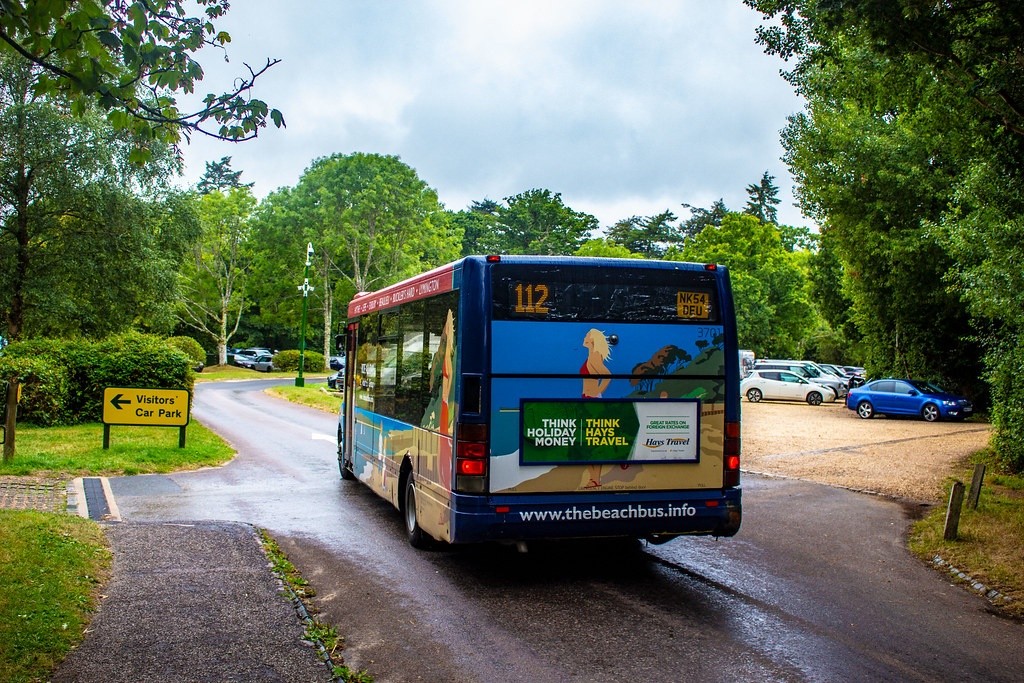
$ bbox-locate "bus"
[336,254,743,550]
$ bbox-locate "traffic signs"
[103,386,190,427]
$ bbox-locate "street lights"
[296,242,315,387]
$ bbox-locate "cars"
[330,356,345,371]
[846,376,973,422]
[739,369,836,406]
[225,346,281,374]
[328,366,365,393]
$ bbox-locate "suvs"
[739,348,867,399]
